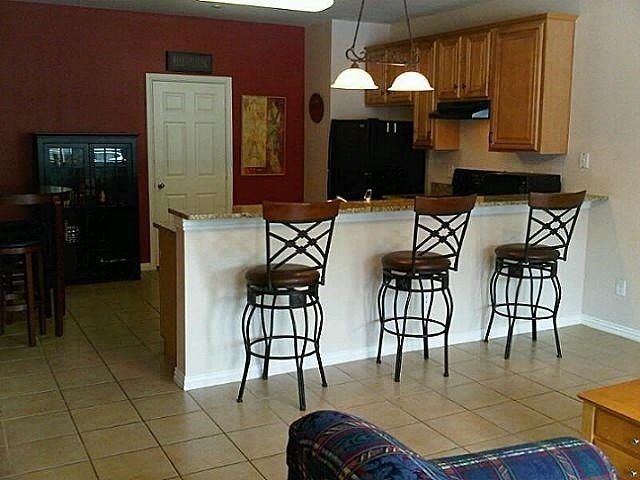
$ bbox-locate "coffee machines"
[451,166,506,195]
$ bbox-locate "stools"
[0,233,46,347]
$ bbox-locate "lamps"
[330,0,435,92]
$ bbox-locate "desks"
[0,193,66,337]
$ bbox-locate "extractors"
[427,101,491,121]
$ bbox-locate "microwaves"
[490,170,562,195]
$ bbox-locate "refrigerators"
[327,117,425,201]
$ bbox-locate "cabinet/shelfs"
[364,39,414,106]
[578,394,640,480]
[488,12,578,155]
[412,37,460,151]
[434,23,492,102]
[31,131,142,285]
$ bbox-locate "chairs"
[286,409,616,480]
[237,200,342,411]
[485,190,586,359]
[376,194,479,382]
